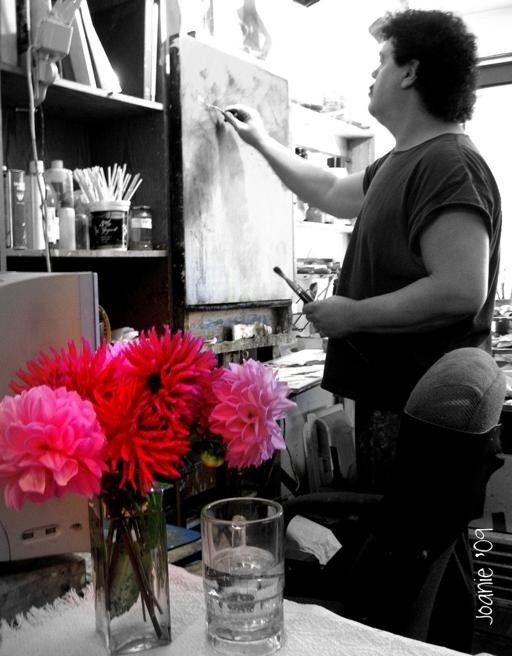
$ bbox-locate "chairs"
[257,347,510,648]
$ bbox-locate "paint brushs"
[73,163,143,202]
[274,267,313,302]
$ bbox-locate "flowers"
[2,325,303,638]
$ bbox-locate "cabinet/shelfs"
[0,0,168,260]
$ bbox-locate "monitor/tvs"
[0,270,101,562]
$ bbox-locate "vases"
[80,482,172,656]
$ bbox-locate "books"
[1,1,162,102]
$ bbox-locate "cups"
[89,202,129,249]
[199,496,287,654]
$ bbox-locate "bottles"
[128,205,155,251]
[30,160,76,250]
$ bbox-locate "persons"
[219,9,502,651]
